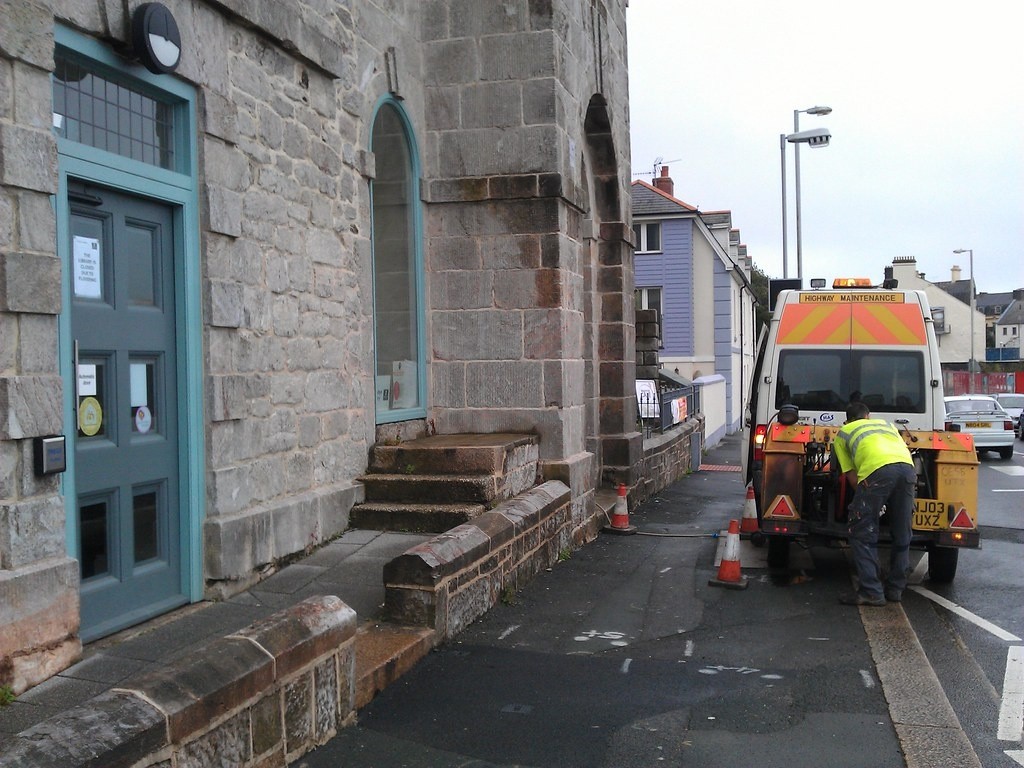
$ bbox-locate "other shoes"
[884,592,901,602]
[839,588,886,606]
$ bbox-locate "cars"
[943,393,1023,458]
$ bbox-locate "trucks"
[742,277,981,586]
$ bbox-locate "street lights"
[953,248,977,395]
[780,105,833,289]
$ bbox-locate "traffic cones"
[602,483,638,536]
[738,485,759,539]
[708,518,749,589]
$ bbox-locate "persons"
[833,402,916,606]
